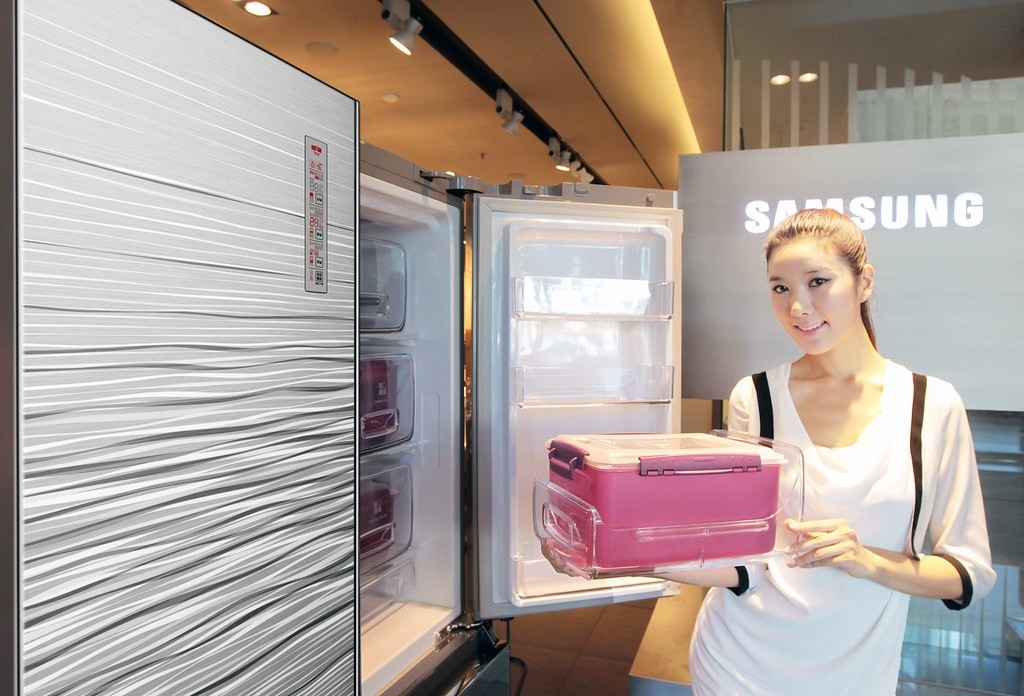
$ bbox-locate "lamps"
[390,14,424,56]
[555,148,572,172]
[502,112,526,136]
[577,165,595,185]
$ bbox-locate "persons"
[542,209,998,696]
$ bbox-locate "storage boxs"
[533,429,803,582]
[350,229,415,575]
